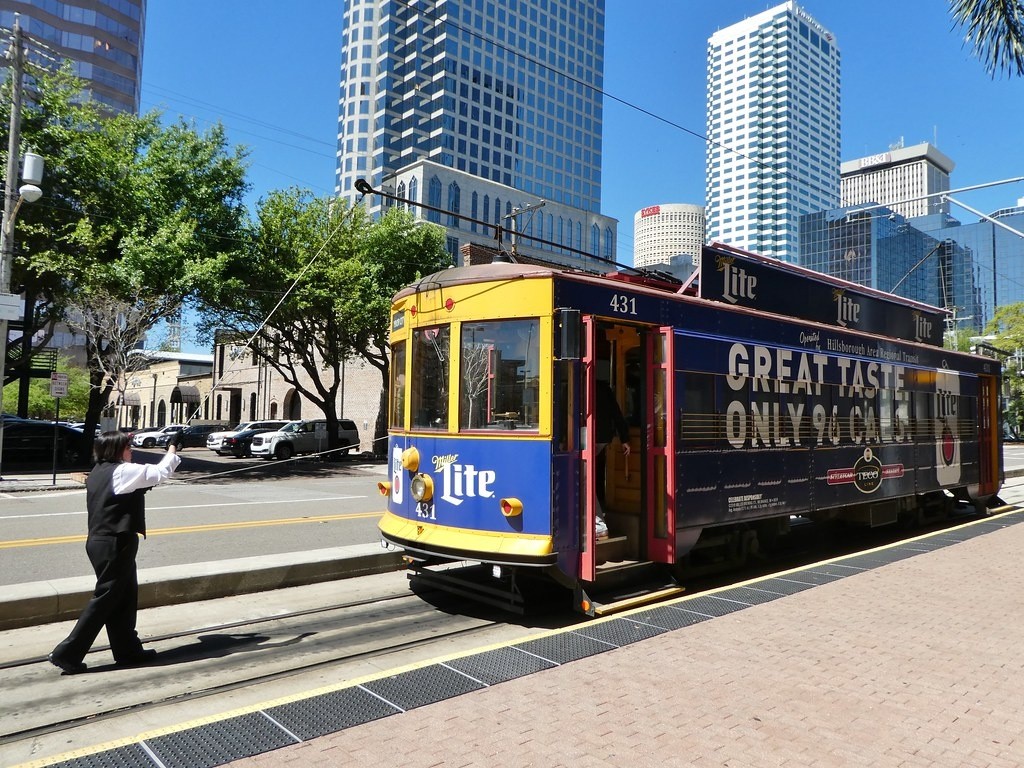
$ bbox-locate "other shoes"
[595,528,609,541]
[46,653,88,674]
[143,649,157,659]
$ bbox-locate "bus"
[354,179,1007,622]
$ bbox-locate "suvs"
[0,415,360,473]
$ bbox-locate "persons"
[595,376,631,541]
[48,431,184,674]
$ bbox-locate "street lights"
[0,151,45,479]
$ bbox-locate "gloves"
[168,429,184,448]
